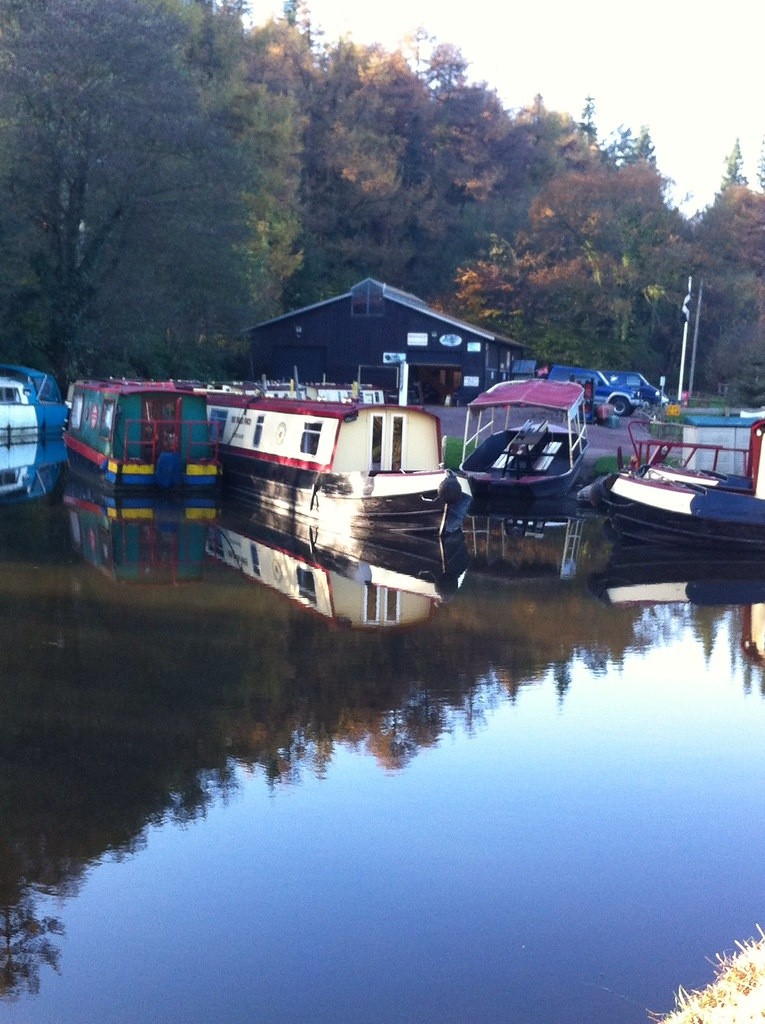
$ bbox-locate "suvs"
[546,365,643,417]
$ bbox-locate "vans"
[601,371,661,403]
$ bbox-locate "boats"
[206,490,473,631]
[1,365,70,436]
[462,491,589,592]
[62,478,222,592]
[459,378,591,501]
[65,378,222,492]
[206,393,462,523]
[588,535,765,665]
[1,436,68,503]
[611,419,765,540]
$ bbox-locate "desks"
[502,419,550,480]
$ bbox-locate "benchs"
[491,439,524,468]
[533,441,563,473]
